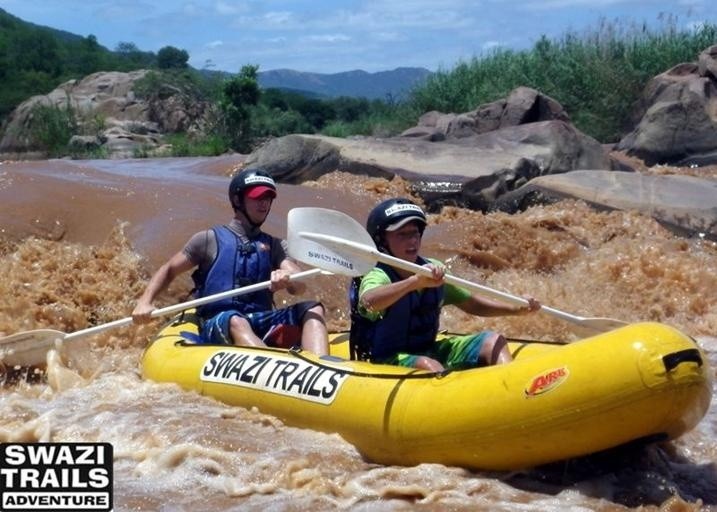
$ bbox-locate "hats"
[243,185,277,199]
[383,215,427,233]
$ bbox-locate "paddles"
[287,208,631,331]
[0,269,340,367]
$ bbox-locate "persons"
[349,198,541,372]
[132,168,330,356]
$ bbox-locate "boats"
[139,306,712,476]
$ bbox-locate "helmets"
[366,198,426,238]
[228,168,276,201]
